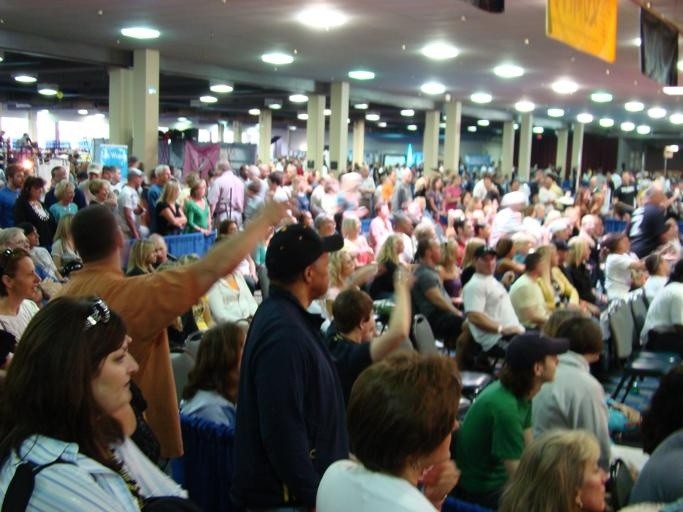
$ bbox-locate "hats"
[267,223,344,272]
[505,332,569,367]
[128,168,146,177]
[87,164,103,175]
[475,245,497,258]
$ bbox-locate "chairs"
[391,338,472,411]
[170,347,200,403]
[608,299,677,376]
[630,293,680,363]
[185,329,209,359]
[411,314,492,394]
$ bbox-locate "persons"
[1,293,146,511]
[533,318,612,472]
[497,428,609,512]
[49,195,302,458]
[314,346,463,511]
[451,329,572,510]
[639,367,683,451]
[2,126,682,399]
[629,427,683,512]
[234,222,350,512]
[157,320,248,510]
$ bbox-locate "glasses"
[81,295,110,331]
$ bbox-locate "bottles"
[630,379,639,395]
[595,279,602,305]
